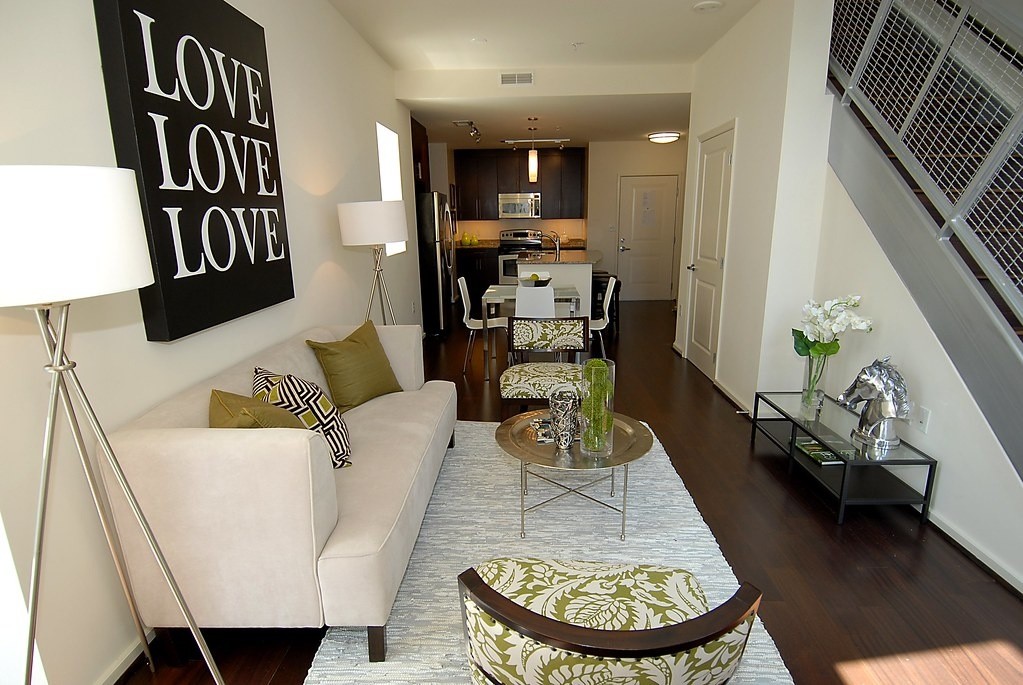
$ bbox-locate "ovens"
[498,248,542,284]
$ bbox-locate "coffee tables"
[496,409,653,541]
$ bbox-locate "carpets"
[304,418,797,684]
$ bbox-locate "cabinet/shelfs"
[411,116,429,184]
[455,249,499,298]
[453,147,583,221]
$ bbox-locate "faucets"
[538,230,560,252]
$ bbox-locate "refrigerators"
[419,192,454,331]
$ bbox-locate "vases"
[800,354,829,409]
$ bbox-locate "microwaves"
[498,193,541,218]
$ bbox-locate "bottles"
[550,235,556,245]
[560,232,569,244]
[570,302,574,312]
[462,232,478,245]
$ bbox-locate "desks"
[750,389,938,527]
[481,284,581,381]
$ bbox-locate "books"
[529,412,590,444]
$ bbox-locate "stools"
[592,268,622,335]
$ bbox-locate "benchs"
[95,322,457,662]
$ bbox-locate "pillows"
[208,389,306,430]
[253,366,352,468]
[305,319,404,414]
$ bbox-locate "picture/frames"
[449,184,456,235]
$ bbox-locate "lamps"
[0,163,227,684]
[648,132,680,144]
[527,116,538,182]
[338,200,410,325]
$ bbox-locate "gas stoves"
[499,228,542,248]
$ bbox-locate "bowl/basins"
[516,277,552,287]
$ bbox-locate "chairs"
[457,556,763,685]
[457,277,514,375]
[576,276,616,364]
[500,286,587,410]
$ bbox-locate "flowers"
[792,294,873,403]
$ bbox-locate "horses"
[835,357,909,449]
